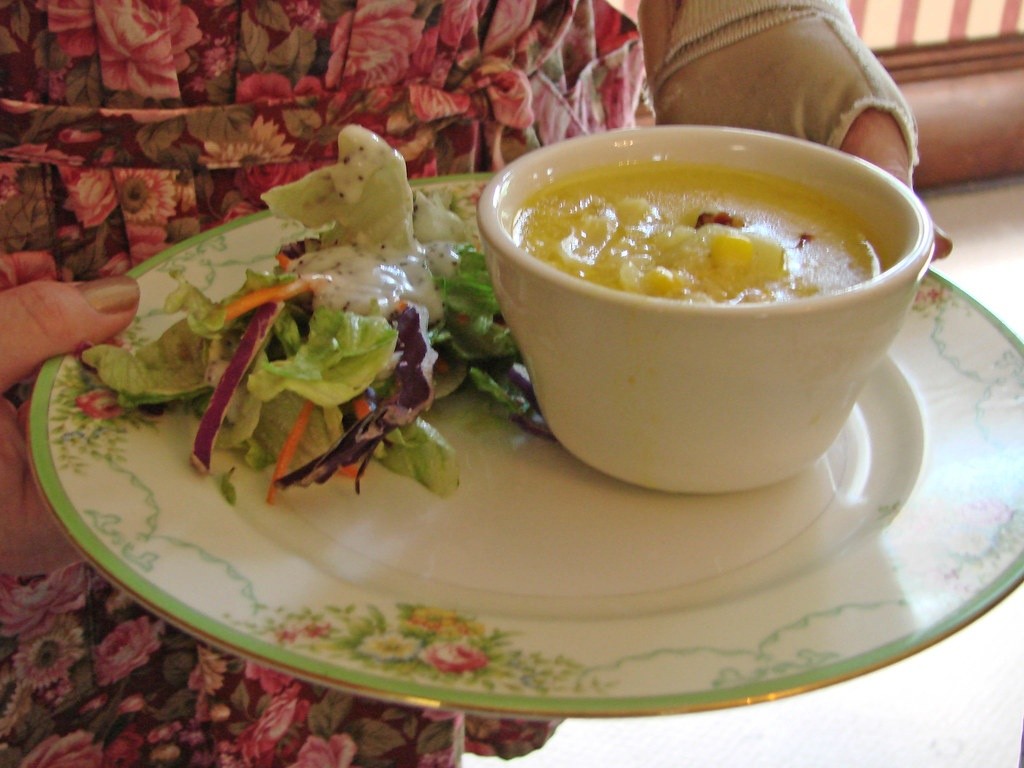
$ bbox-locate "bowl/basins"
[477,123,936,494]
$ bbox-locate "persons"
[0,0,951,768]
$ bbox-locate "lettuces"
[81,124,567,499]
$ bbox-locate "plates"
[26,171,1023,719]
